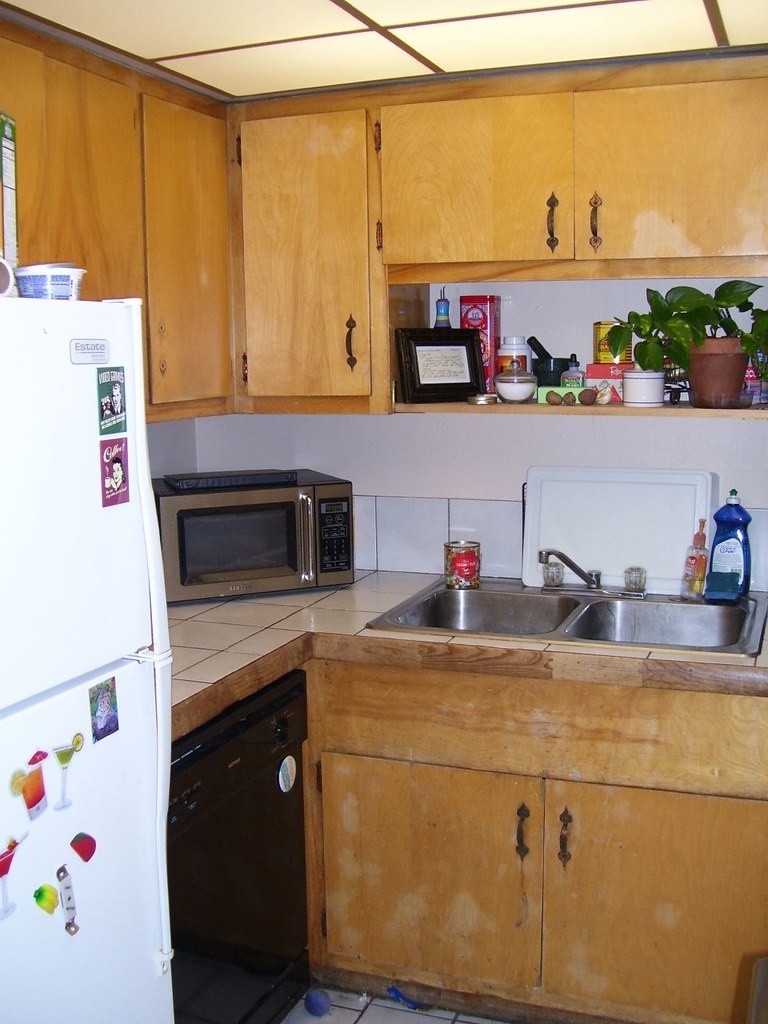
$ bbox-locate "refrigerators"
[1,296,174,1024]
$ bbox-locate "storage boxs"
[585,363,635,403]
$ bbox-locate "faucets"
[537,549,602,589]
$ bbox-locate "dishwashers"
[167,670,310,1023]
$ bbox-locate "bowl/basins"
[494,361,537,403]
[15,263,88,301]
[622,371,665,407]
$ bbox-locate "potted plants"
[606,279,768,406]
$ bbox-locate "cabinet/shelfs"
[314,750,768,1024]
[0,38,768,409]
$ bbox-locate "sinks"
[365,580,586,639]
[564,591,768,658]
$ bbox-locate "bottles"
[433,299,451,329]
[560,354,585,388]
[498,336,532,375]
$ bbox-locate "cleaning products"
[680,488,752,604]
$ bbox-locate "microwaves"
[151,469,355,606]
